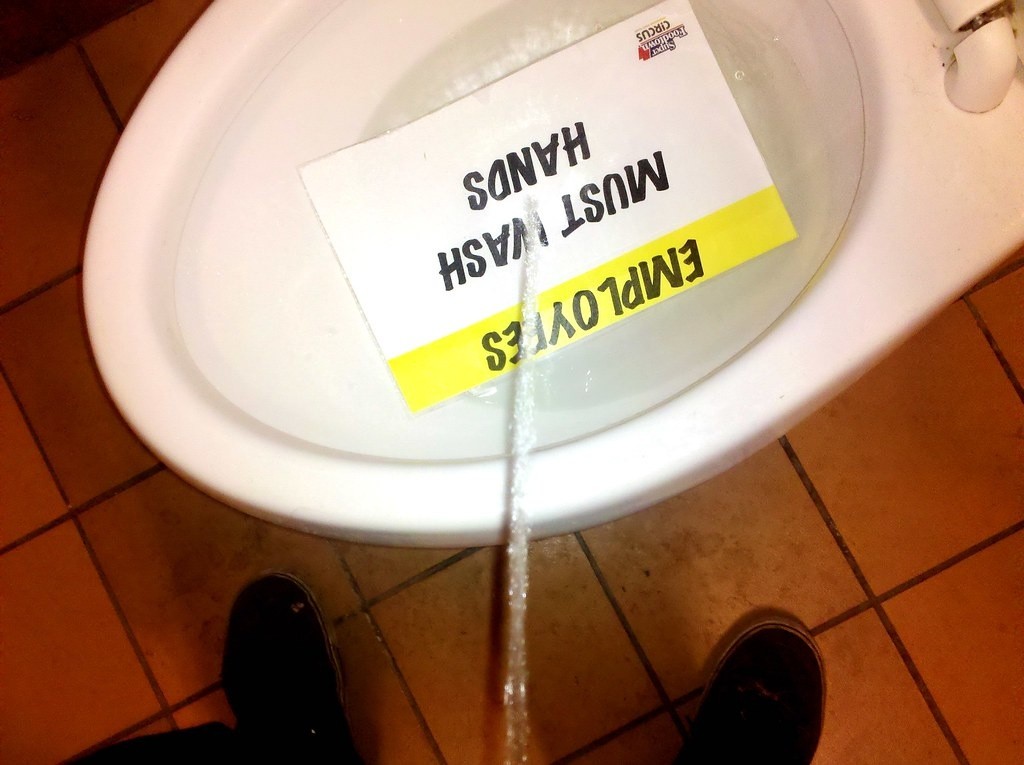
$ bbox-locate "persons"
[59,572,832,765]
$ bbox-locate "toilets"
[81,2,1024,549]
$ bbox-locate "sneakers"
[669,613,827,764]
[218,573,362,764]
